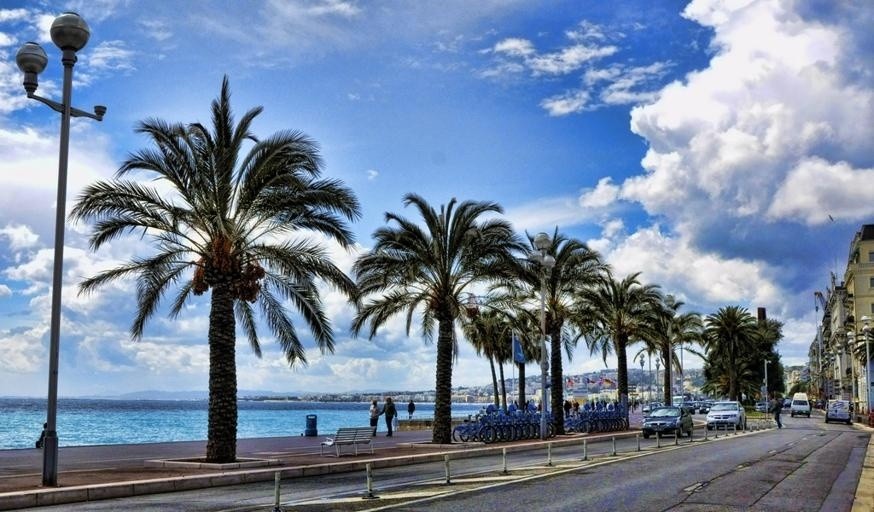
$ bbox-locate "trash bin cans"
[305,414,317,437]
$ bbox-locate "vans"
[791,392,811,417]
[825,399,854,425]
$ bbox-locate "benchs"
[319,425,376,457]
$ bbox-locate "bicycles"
[453,411,630,443]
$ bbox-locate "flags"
[512,331,526,363]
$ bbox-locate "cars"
[642,394,717,415]
[707,400,747,430]
[641,406,693,439]
[755,398,792,413]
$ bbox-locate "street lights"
[820,315,871,414]
[639,353,645,399]
[17,12,108,486]
[655,357,660,401]
[529,232,558,441]
[764,358,772,420]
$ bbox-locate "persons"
[513,398,640,420]
[368,398,397,438]
[36,423,47,448]
[768,395,782,428]
[407,398,418,418]
